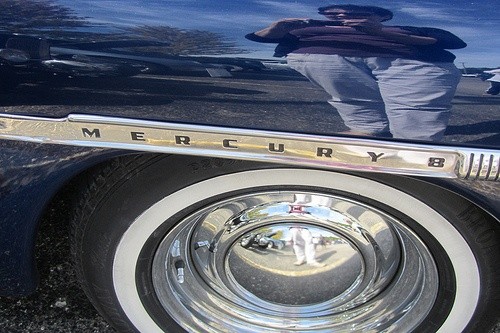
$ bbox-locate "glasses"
[318,11,377,17]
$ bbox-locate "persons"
[482,66,500,96]
[244,3,468,166]
[287,204,326,268]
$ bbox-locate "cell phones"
[306,20,346,28]
